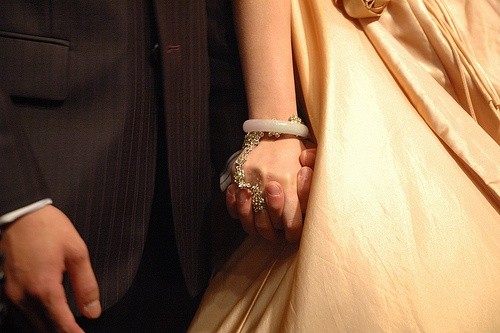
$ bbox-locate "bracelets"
[234,114,305,213]
[240,119,309,138]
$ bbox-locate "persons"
[227,2,500,333]
[1,1,318,333]
